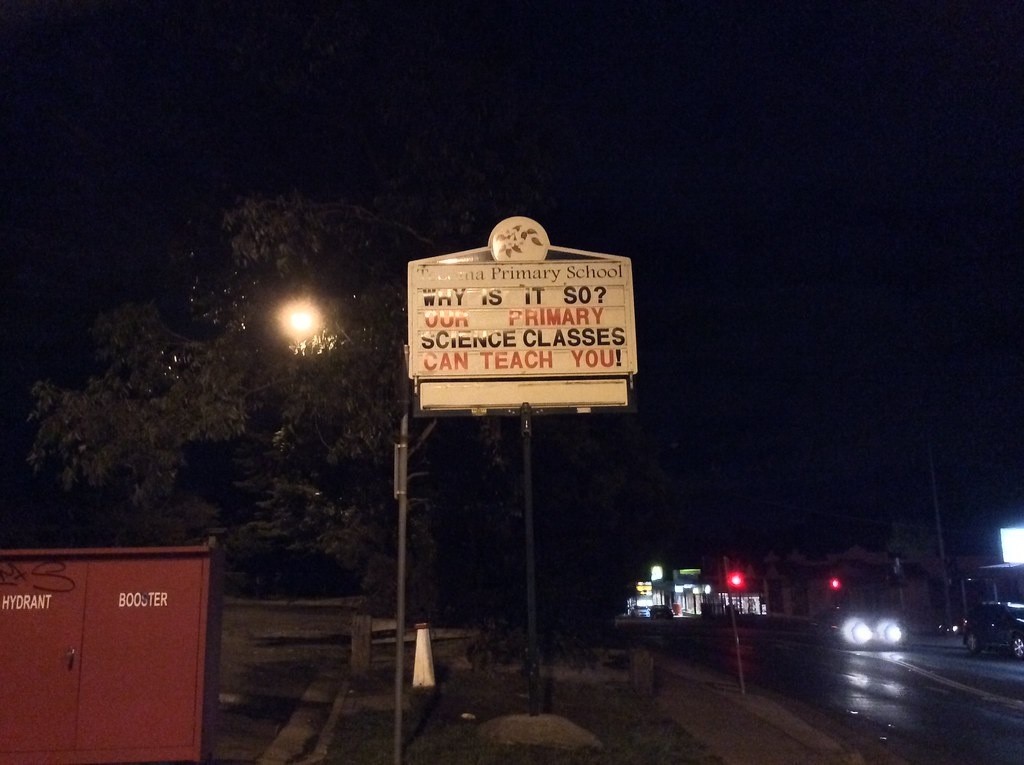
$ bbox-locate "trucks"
[1,543,220,764]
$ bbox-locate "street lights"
[274,297,413,765]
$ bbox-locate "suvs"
[962,601,1023,660]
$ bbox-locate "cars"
[649,605,674,619]
[822,609,906,649]
[632,605,650,619]
